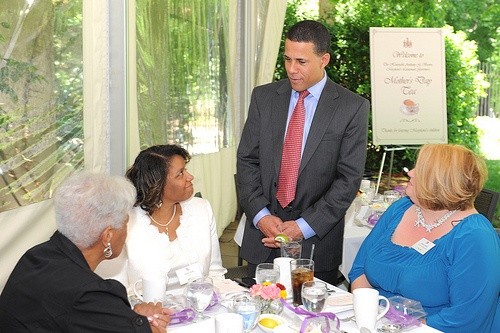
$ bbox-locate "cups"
[281,236,303,265]
[215,313,243,333]
[274,257,296,298]
[384,191,399,209]
[353,288,390,331]
[255,263,280,286]
[301,281,328,314]
[290,259,314,305]
[134,273,166,303]
[361,180,370,188]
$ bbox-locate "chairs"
[474,189,499,223]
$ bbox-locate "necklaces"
[414,206,459,232]
[148,204,176,231]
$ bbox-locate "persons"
[348,143,500,333]
[235,20,369,277]
[0,170,173,333]
[125,144,224,295]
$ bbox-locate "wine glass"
[187,276,213,317]
[230,293,261,333]
[370,181,378,201]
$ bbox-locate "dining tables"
[128,276,445,333]
[338,194,403,284]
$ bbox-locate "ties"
[276,90,311,209]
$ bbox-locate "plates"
[326,293,353,306]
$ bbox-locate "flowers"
[248,282,287,310]
